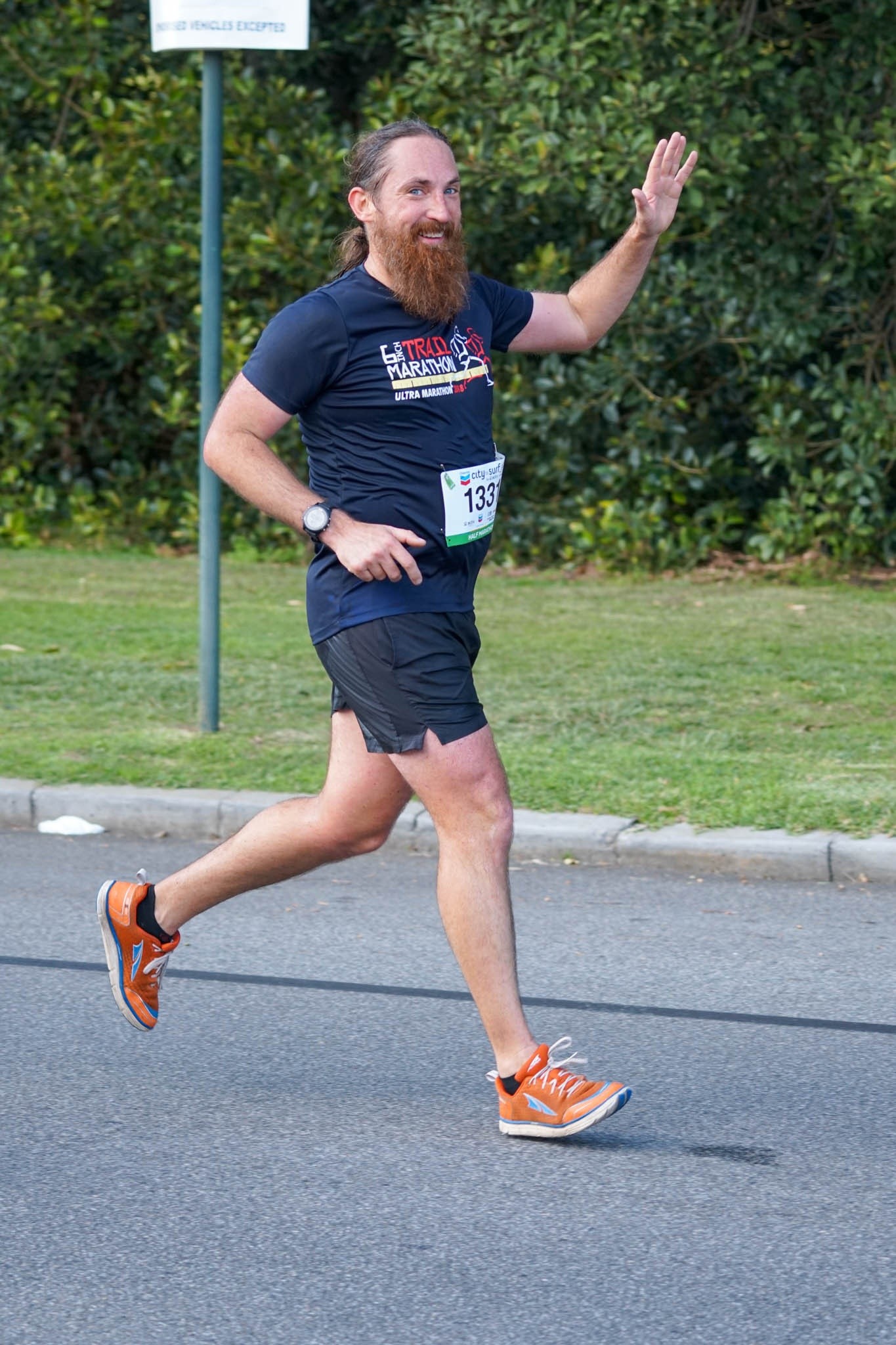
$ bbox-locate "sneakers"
[485,1037,633,1140]
[96,868,181,1034]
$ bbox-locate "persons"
[95,121,702,1136]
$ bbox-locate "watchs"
[302,500,339,543]
